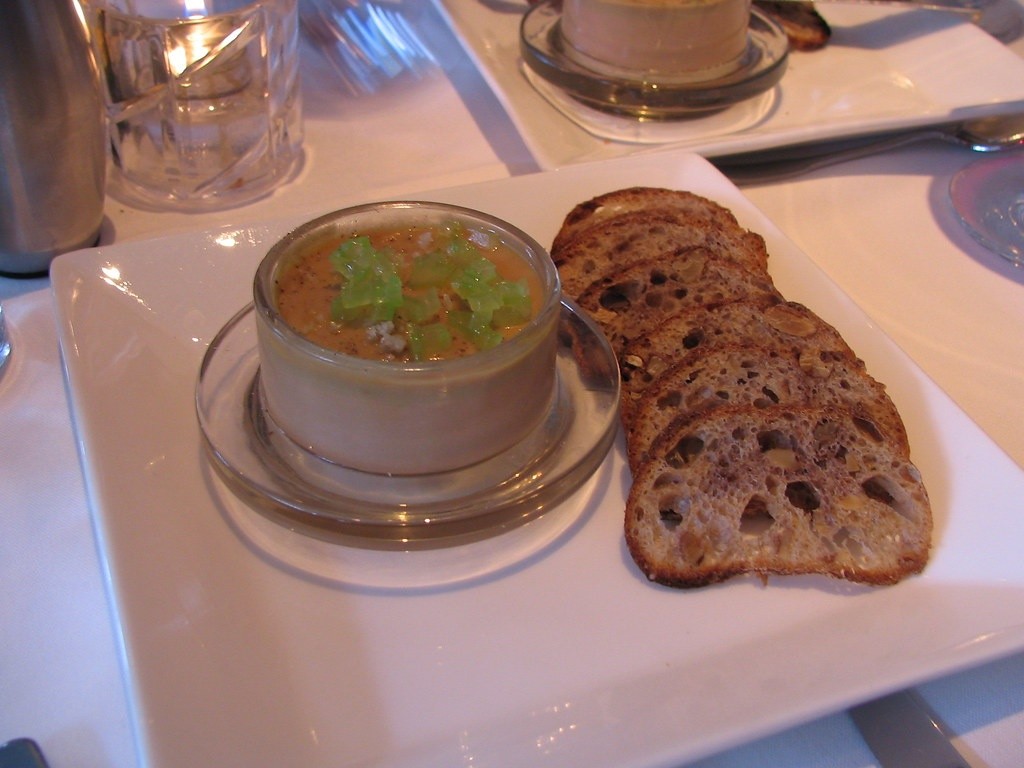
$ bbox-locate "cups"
[559,0,755,76]
[0,0,108,276]
[250,197,565,486]
[69,0,307,208]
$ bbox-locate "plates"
[518,0,792,118]
[48,148,1024,768]
[428,0,1024,174]
[192,291,625,541]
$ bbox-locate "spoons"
[715,111,1024,173]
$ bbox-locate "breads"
[550,207,758,344]
[567,247,789,382]
[624,338,911,477]
[622,399,935,587]
[548,186,765,266]
[619,298,868,437]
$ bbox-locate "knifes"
[846,690,975,768]
